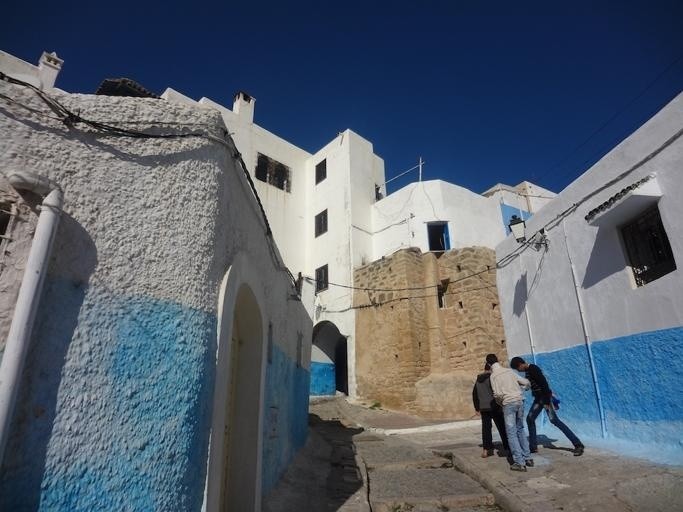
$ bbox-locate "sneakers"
[530,448,538,453]
[483,450,488,457]
[526,459,534,467]
[510,462,527,471]
[573,445,584,456]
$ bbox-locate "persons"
[510,357,585,456]
[487,354,534,471]
[472,362,511,458]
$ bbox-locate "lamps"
[508,215,547,247]
